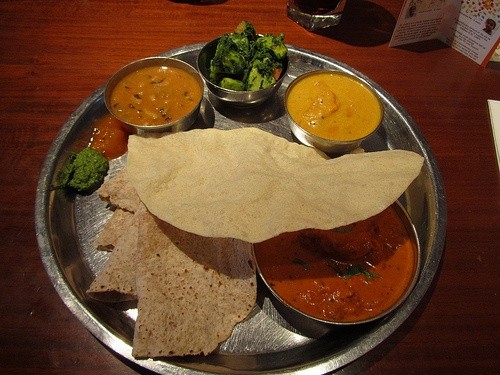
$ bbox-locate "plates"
[34,41,449,375]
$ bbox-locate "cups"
[286,0,348,32]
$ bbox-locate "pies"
[83,128,425,358]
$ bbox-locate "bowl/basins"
[102,56,204,135]
[197,32,289,108]
[283,69,384,157]
[250,186,422,339]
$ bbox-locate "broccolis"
[208,19,287,92]
